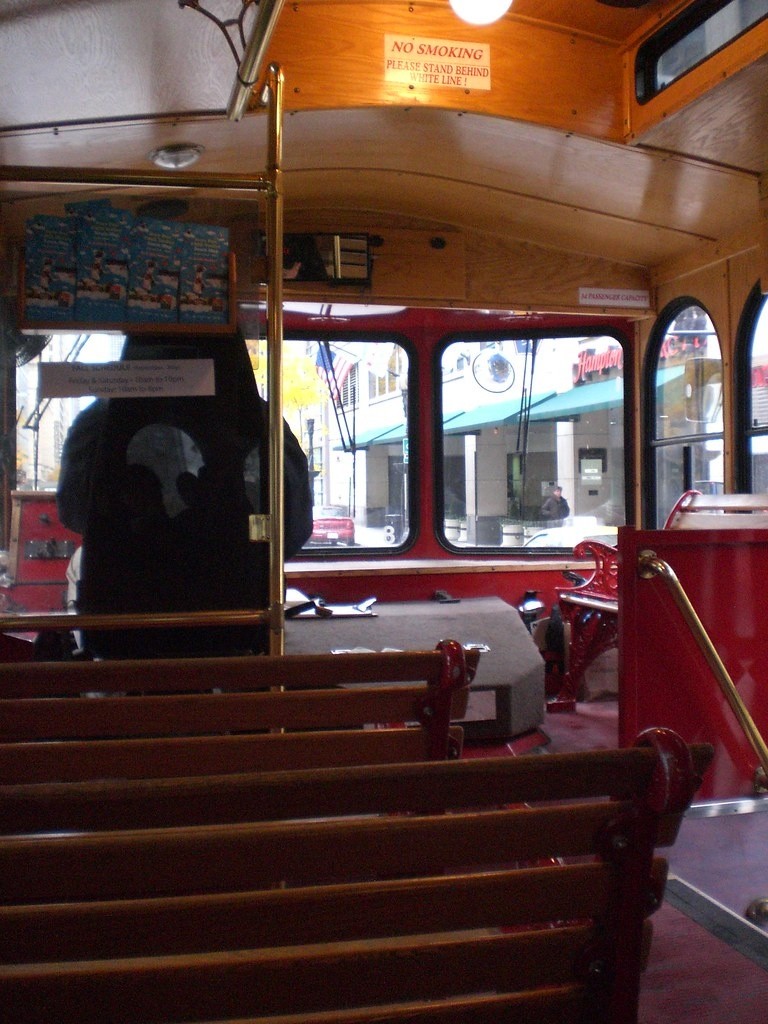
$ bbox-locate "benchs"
[0,638,716,1024]
[546,490,768,711]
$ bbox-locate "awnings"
[331,366,685,451]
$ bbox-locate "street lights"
[304,405,321,509]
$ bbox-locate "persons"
[520,464,542,521]
[57,317,313,658]
[450,481,466,515]
[262,234,305,283]
[541,486,569,529]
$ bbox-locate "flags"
[315,345,361,401]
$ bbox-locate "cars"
[307,506,356,547]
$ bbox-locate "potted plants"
[444,513,467,543]
[501,518,545,546]
